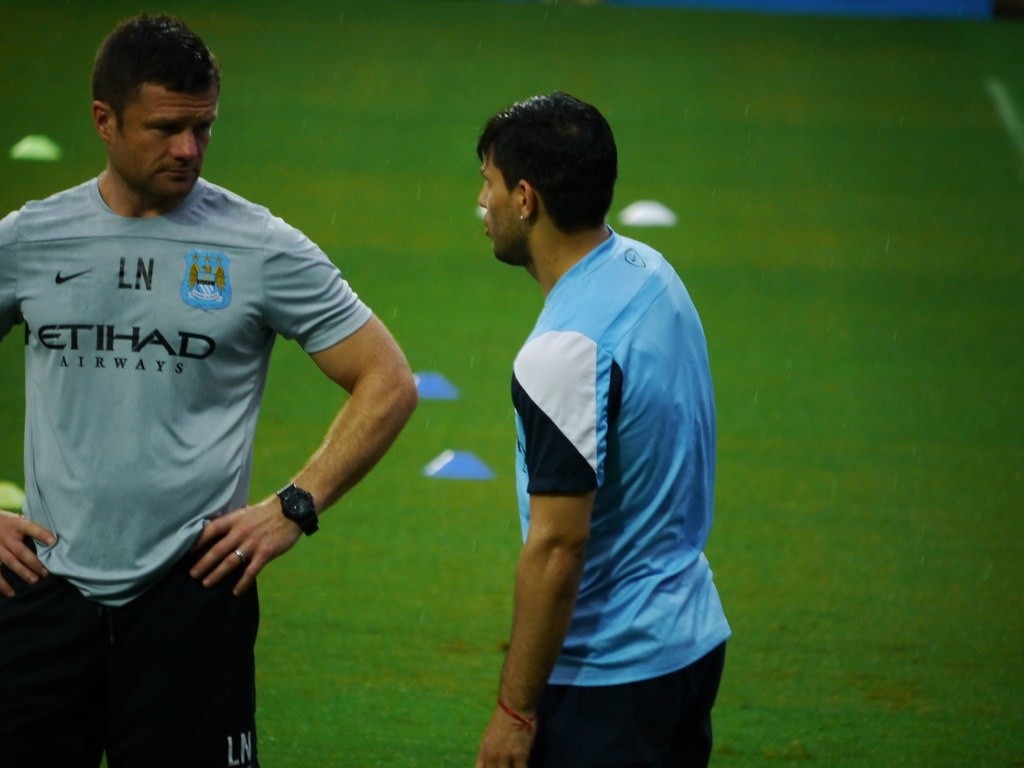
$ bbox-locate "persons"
[471,92,729,768]
[0,13,415,766]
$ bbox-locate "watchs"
[274,479,321,540]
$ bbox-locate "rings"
[234,551,245,564]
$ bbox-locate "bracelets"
[496,700,538,732]
